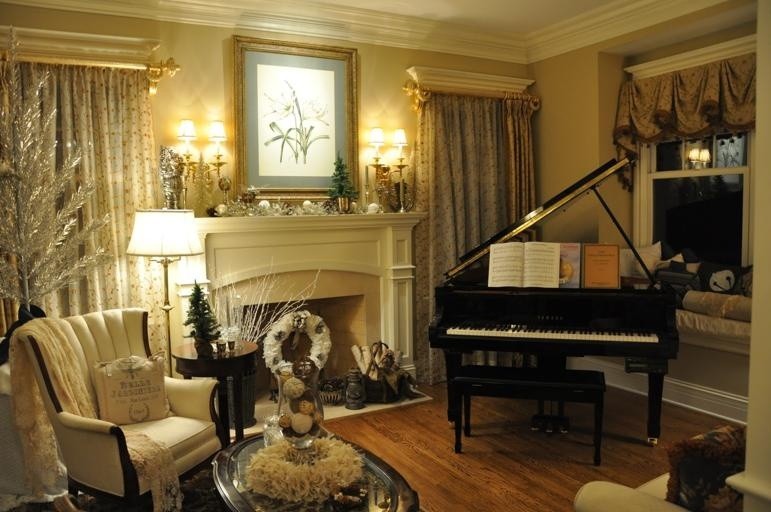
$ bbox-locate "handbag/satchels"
[360,341,404,404]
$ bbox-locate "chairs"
[6,310,229,509]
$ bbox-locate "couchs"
[573,422,747,510]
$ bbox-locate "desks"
[171,338,261,431]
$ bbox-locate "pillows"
[615,239,752,321]
[663,422,747,509]
[90,349,178,426]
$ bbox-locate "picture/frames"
[233,34,363,202]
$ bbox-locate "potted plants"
[321,148,359,212]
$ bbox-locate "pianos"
[428,157,679,446]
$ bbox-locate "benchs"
[444,363,608,468]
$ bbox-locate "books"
[487,243,561,290]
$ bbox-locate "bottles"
[264,415,285,447]
[275,364,324,466]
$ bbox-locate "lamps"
[368,123,392,214]
[392,127,417,210]
[688,144,701,169]
[207,116,229,212]
[699,149,712,170]
[174,114,203,210]
[121,205,206,381]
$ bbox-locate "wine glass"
[216,341,236,353]
[220,354,236,358]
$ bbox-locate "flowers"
[263,308,335,379]
[260,71,334,166]
